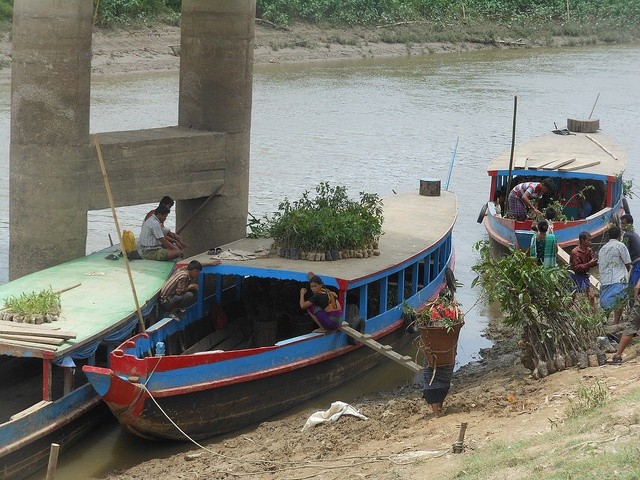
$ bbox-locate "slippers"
[312,329,327,332]
[207,246,216,254]
[214,247,224,254]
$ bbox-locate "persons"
[423,368,459,419]
[158,260,221,323]
[584,180,607,213]
[494,190,505,215]
[598,226,632,311]
[507,179,556,220]
[603,260,640,363]
[570,231,596,292]
[545,208,556,234]
[529,222,558,267]
[144,195,189,251]
[299,272,343,335]
[137,206,183,260]
[620,213,640,296]
[254,277,291,302]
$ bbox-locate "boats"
[0,238,176,480]
[482,92,627,266]
[82,135,459,441]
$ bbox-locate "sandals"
[602,356,622,363]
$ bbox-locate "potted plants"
[246,178,386,261]
[470,236,617,378]
[0,283,62,325]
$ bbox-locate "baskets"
[417,321,465,367]
[252,319,278,347]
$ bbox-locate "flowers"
[399,292,464,333]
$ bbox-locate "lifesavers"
[403,309,419,333]
[612,211,621,225]
[445,268,457,292]
[622,198,631,214]
[347,315,367,346]
[477,204,487,224]
